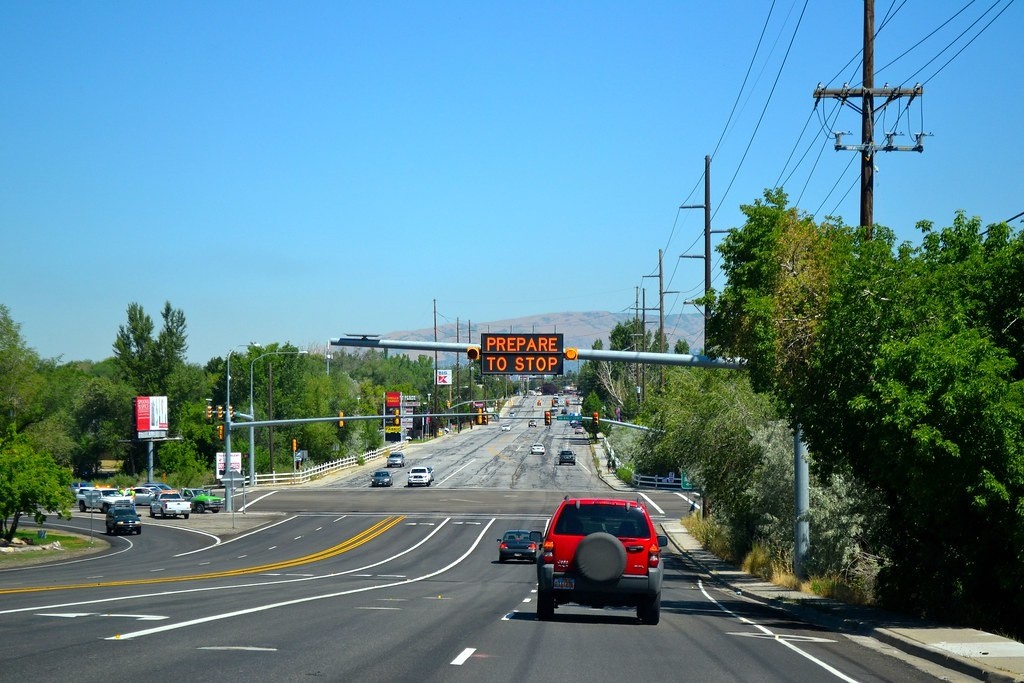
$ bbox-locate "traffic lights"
[566,349,577,360]
[207,406,213,420]
[229,405,234,418]
[483,416,488,425]
[468,348,480,360]
[545,411,551,426]
[218,406,223,419]
[593,413,599,426]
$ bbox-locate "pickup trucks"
[180,487,224,514]
[150,492,193,519]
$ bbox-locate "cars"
[496,530,539,565]
[550,391,567,414]
[371,470,393,487]
[428,467,434,481]
[126,483,172,506]
[501,424,511,431]
[531,443,545,454]
[570,420,587,435]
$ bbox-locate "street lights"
[222,343,261,513]
[447,385,469,431]
[250,351,310,486]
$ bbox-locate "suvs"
[408,467,431,487]
[529,420,537,427]
[528,495,668,625]
[558,450,577,464]
[105,507,142,535]
[386,452,405,467]
[77,487,134,513]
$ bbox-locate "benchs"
[567,517,637,536]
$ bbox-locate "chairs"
[508,535,514,539]
[524,536,529,539]
